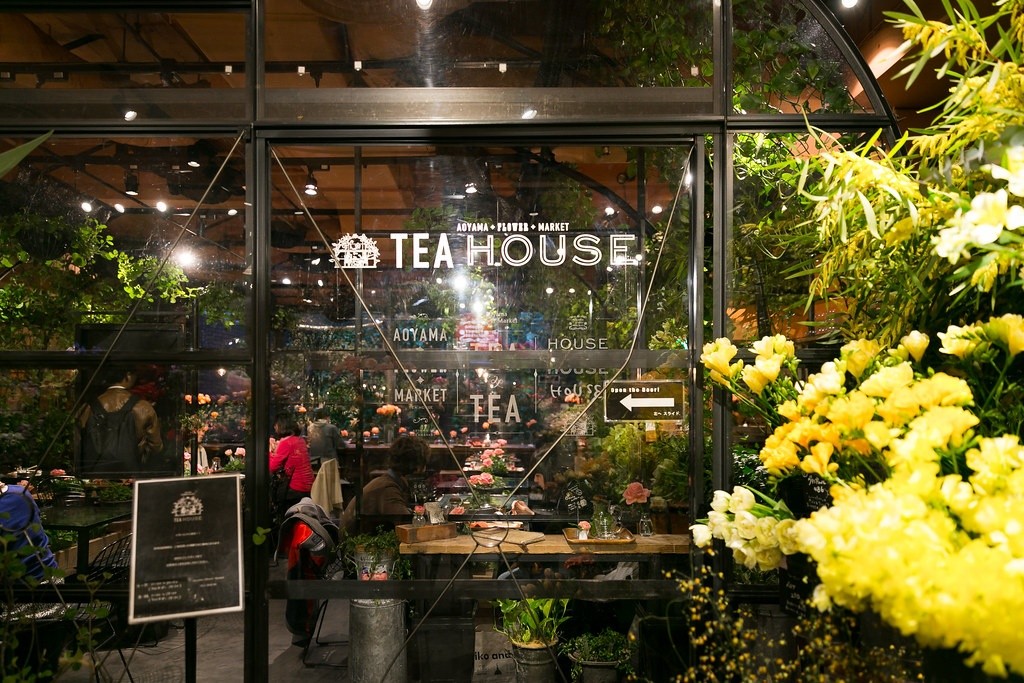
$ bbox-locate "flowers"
[0,393,653,522]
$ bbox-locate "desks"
[463,466,524,478]
[399,534,690,683]
[453,478,528,488]
[41,502,131,575]
[438,494,528,531]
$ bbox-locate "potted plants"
[488,595,571,683]
[333,526,418,621]
[558,626,640,682]
[0,440,713,683]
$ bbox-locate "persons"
[508,443,581,534]
[340,434,432,639]
[268,410,315,540]
[71,360,164,478]
[0,482,68,675]
[306,408,346,472]
[185,424,211,474]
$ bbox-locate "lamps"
[305,171,317,195]
[126,171,140,195]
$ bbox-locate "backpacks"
[81,393,141,473]
